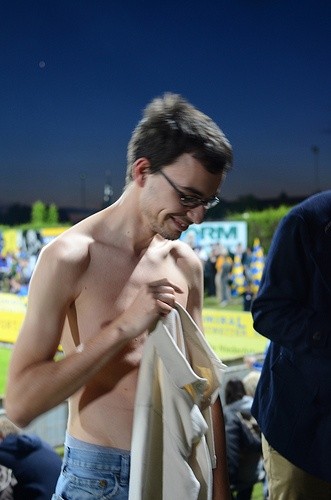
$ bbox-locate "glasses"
[158,169,219,210]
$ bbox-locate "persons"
[192,238,265,311]
[223,339,272,500]
[4,93,234,500]
[251,191,331,500]
[0,416,62,500]
[1,228,38,296]
